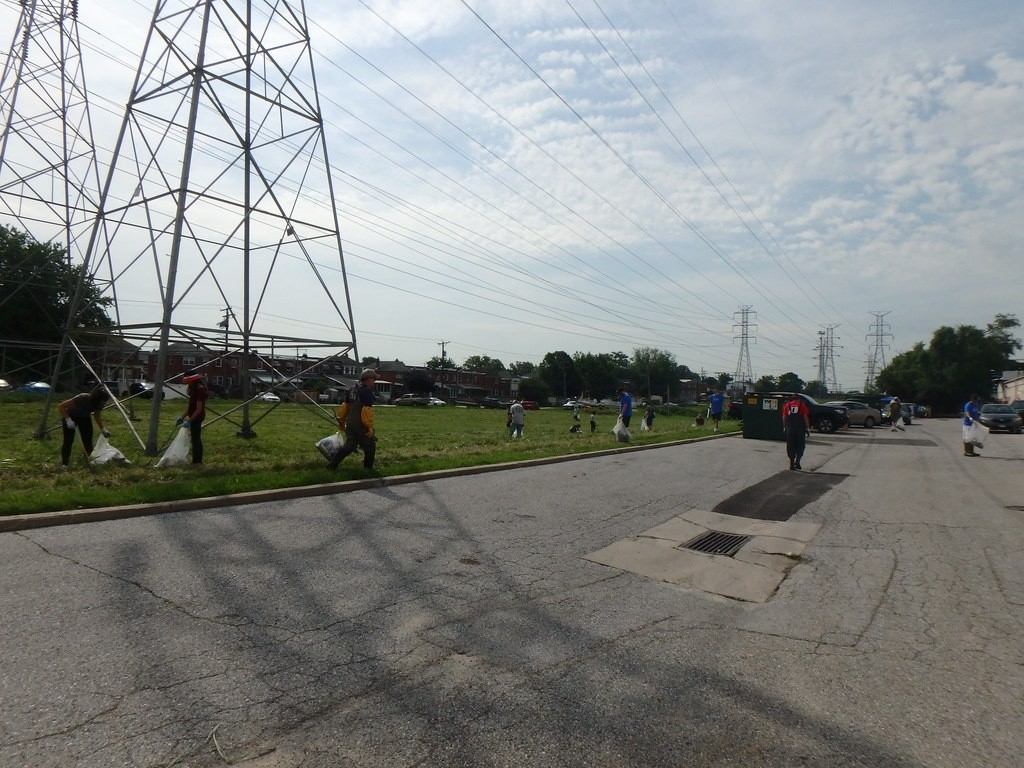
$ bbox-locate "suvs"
[741,390,851,434]
[868,394,912,424]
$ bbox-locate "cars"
[427,397,446,407]
[478,397,541,411]
[253,391,280,402]
[1011,399,1024,426]
[16,382,50,393]
[0,379,12,391]
[122,382,165,400]
[823,400,883,429]
[561,399,605,408]
[726,400,744,420]
[977,403,1023,433]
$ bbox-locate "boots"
[963,443,980,456]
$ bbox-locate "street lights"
[295,346,309,386]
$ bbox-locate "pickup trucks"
[392,393,430,407]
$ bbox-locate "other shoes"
[714,428,719,432]
[326,462,337,470]
[891,429,897,432]
[794,461,801,469]
[790,466,795,470]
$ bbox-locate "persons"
[175,370,209,464]
[642,402,654,430]
[326,368,377,472]
[57,390,111,467]
[570,424,580,433]
[618,387,632,431]
[590,411,597,432]
[889,396,901,432]
[708,386,725,432]
[781,395,811,470]
[506,400,525,440]
[573,405,580,422]
[695,414,704,427]
[962,394,981,457]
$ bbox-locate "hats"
[642,402,646,407]
[513,400,516,403]
[970,394,978,402]
[894,397,898,400]
[361,369,381,379]
[0,0,1014,587]
[791,394,799,399]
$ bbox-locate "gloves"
[805,428,811,437]
[183,421,191,428]
[66,417,75,428]
[338,422,345,430]
[642,416,645,419]
[970,418,973,421]
[102,428,111,439]
[177,417,184,425]
[366,427,374,438]
[783,428,786,432]
